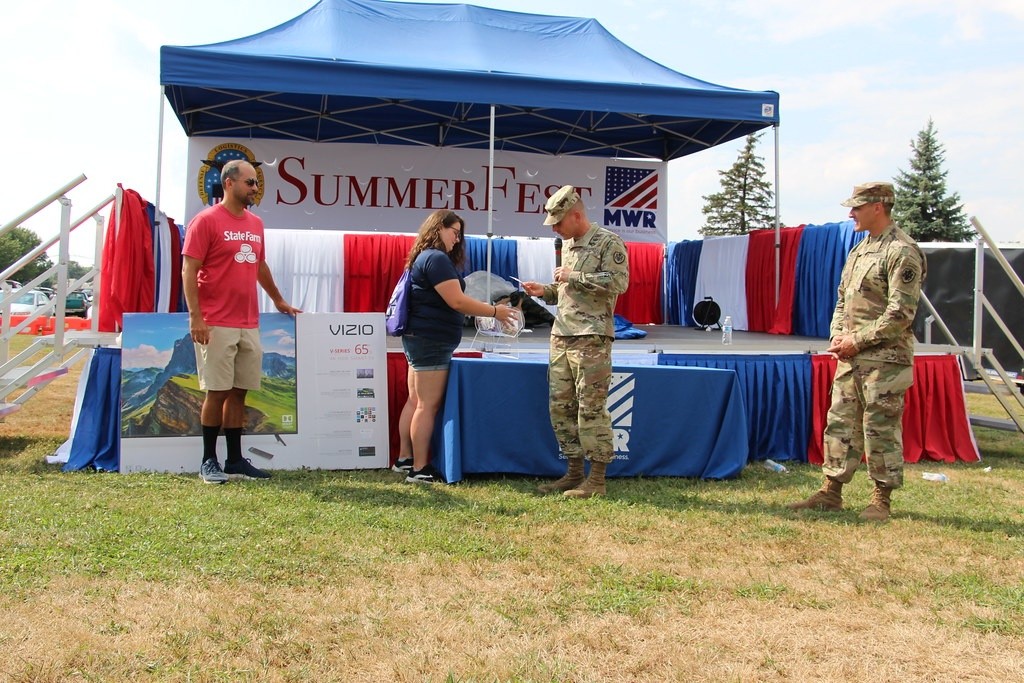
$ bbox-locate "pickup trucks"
[66,291,91,319]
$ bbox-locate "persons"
[392,209,520,487]
[785,183,927,521]
[520,185,629,499]
[181,160,304,485]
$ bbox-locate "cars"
[0,280,56,316]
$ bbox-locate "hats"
[841,181,895,207]
[543,185,580,226]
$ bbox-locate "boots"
[563,462,607,498]
[858,482,893,521]
[787,475,844,512]
[538,458,586,494]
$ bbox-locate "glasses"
[450,226,461,238]
[230,177,259,187]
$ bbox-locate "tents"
[154,0,781,309]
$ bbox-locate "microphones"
[554,238,562,280]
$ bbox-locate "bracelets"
[493,305,497,317]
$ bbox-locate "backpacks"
[613,313,648,339]
[385,248,425,337]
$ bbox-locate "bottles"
[722,316,733,345]
[922,472,949,483]
[763,458,789,475]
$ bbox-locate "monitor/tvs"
[119,312,298,446]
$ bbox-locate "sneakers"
[391,457,414,474]
[223,458,271,480]
[406,464,448,485]
[199,458,230,484]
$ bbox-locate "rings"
[511,312,514,318]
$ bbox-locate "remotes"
[249,447,274,460]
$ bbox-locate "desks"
[432,356,749,484]
